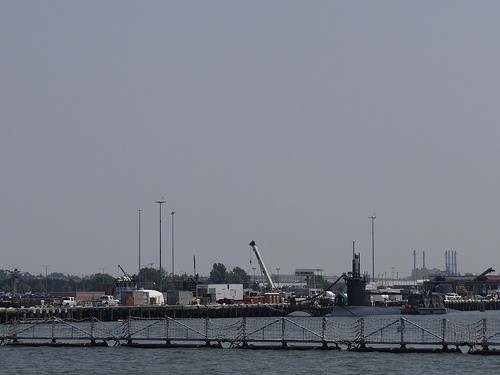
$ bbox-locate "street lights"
[155,200,166,273]
[368,215,377,282]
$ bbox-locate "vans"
[61,297,79,306]
[279,292,297,300]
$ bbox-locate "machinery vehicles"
[248,241,279,293]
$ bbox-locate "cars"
[473,295,484,299]
[444,292,461,300]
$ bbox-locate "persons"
[60,297,63,307]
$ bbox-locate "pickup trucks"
[295,291,336,306]
[91,294,120,307]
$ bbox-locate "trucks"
[133,290,165,306]
